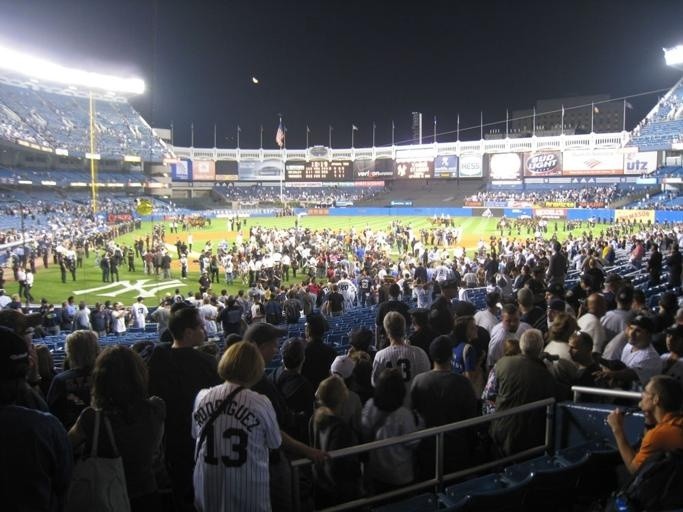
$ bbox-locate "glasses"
[22,328,36,335]
[621,406,641,416]
[573,329,587,338]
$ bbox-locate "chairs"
[33,322,225,374]
[466,285,503,321]
[562,250,682,310]
[264,288,457,380]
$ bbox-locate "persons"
[0,94,683,512]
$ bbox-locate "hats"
[629,315,654,334]
[439,279,458,289]
[243,322,288,348]
[429,334,456,362]
[0,325,29,364]
[330,355,355,379]
[406,308,431,320]
[0,310,42,334]
[548,301,565,311]
[306,312,329,332]
[663,323,683,337]
[577,271,596,287]
[281,337,307,368]
[543,282,566,301]
[587,267,607,278]
[597,356,626,371]
[384,311,406,336]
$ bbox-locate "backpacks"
[602,448,683,512]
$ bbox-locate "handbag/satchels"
[63,405,131,512]
